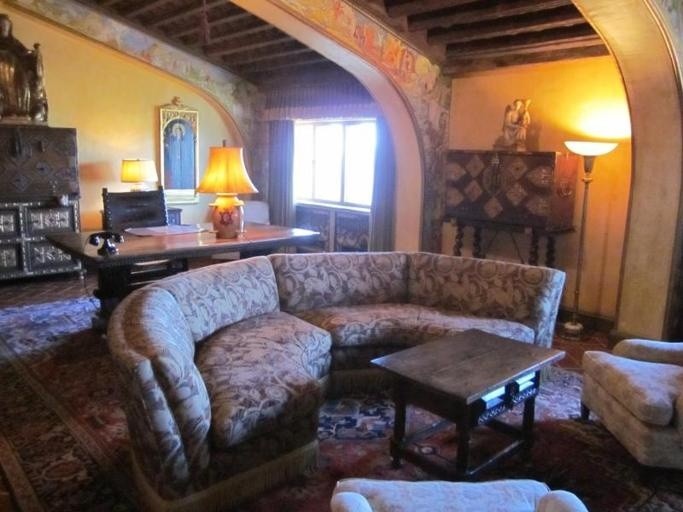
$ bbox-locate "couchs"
[107,256,333,512]
[269,250,566,397]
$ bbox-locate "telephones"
[89,231,124,257]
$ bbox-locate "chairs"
[580,339,683,469]
[331,477,588,512]
[102,186,168,231]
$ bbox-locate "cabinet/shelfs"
[442,149,578,227]
[0,123,84,281]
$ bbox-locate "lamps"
[121,158,159,192]
[195,139,259,240]
[563,140,621,335]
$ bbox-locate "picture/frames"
[160,97,198,204]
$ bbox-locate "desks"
[100,206,182,230]
[440,218,576,268]
[45,221,322,335]
